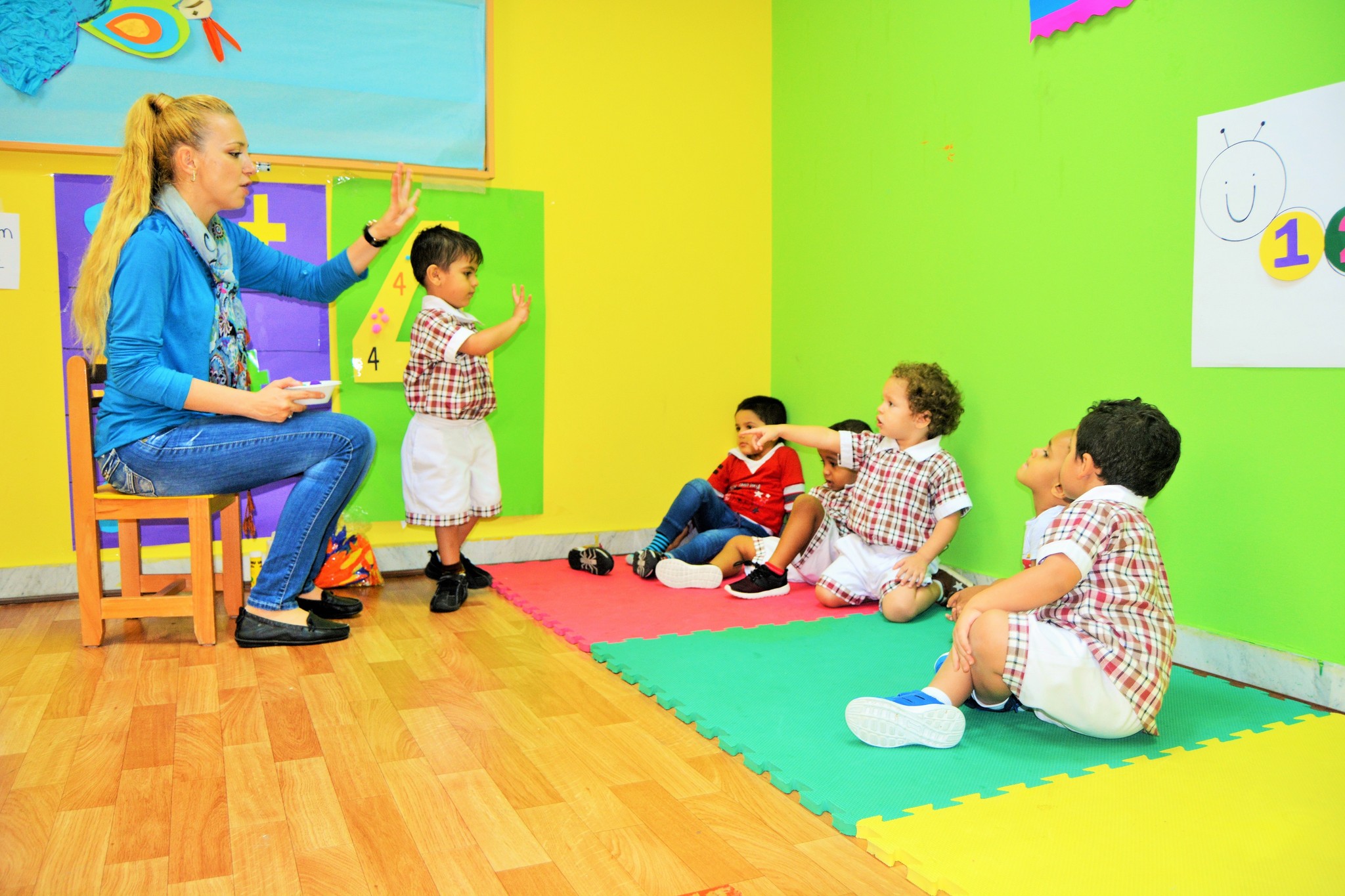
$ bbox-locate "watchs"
[363,220,390,248]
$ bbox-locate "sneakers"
[845,688,966,749]
[934,651,1029,712]
[568,544,614,575]
[424,550,492,589]
[632,550,661,578]
[930,564,977,609]
[430,571,468,612]
[724,560,790,599]
[655,558,723,589]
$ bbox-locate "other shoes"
[235,606,350,648]
[295,589,362,619]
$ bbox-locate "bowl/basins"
[284,380,342,407]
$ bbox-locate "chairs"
[64,356,250,648]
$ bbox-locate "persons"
[625,395,804,579]
[71,93,420,648]
[656,419,873,599]
[741,362,974,623]
[946,429,1077,620]
[401,224,533,613]
[844,397,1183,749]
